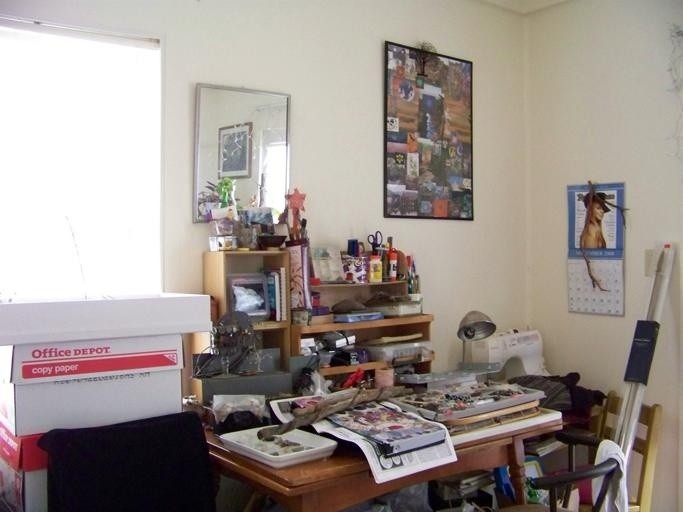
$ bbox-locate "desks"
[201,408,563,512]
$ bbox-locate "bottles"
[369,255,383,283]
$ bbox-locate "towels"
[592,439,629,512]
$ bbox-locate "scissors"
[368,231,382,255]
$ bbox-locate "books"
[441,472,496,498]
[261,270,280,323]
[358,331,423,345]
[520,436,567,458]
[261,267,287,323]
[288,244,313,311]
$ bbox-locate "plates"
[220,423,338,471]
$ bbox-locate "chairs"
[578,391,663,512]
[499,430,618,512]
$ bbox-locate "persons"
[577,191,612,251]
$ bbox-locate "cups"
[341,240,369,284]
[211,220,232,236]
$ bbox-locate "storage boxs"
[0,293,213,512]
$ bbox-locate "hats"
[583,189,612,214]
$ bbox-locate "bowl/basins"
[257,234,288,250]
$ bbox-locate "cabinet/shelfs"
[187,250,434,419]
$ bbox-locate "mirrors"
[458,310,497,375]
[192,83,291,227]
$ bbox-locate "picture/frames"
[218,123,252,179]
[384,41,473,221]
